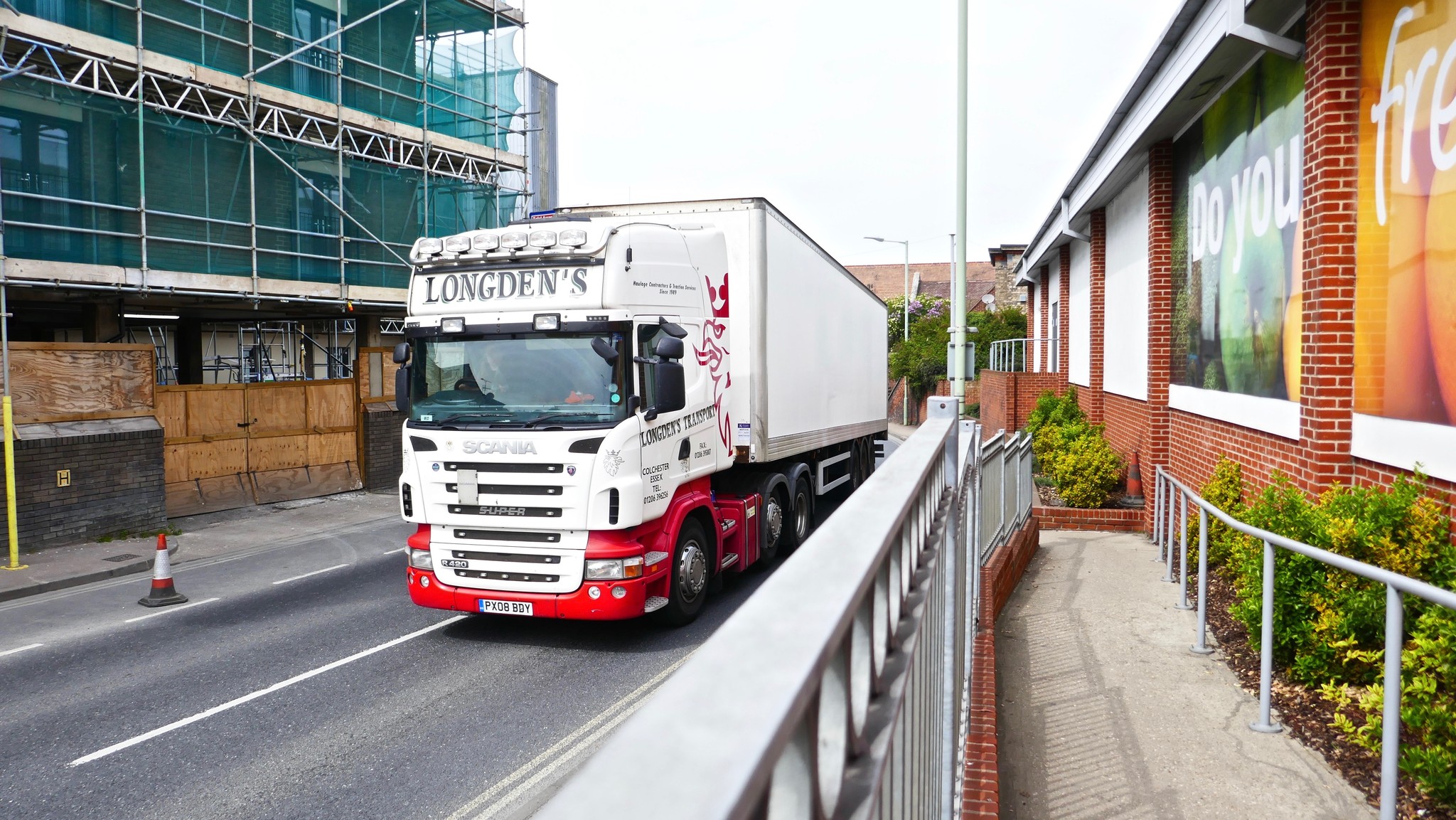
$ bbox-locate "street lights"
[863,236,908,426]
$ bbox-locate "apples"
[1200,18,1305,399]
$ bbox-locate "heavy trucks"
[392,195,889,631]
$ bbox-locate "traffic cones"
[137,534,188,607]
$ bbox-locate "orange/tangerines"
[1281,74,1456,424]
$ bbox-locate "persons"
[459,344,511,393]
